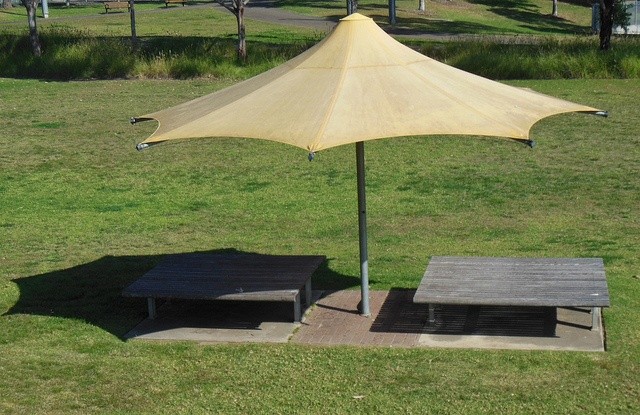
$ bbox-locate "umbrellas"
[131,13,608,317]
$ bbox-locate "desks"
[122,255,326,323]
[414,255,612,330]
